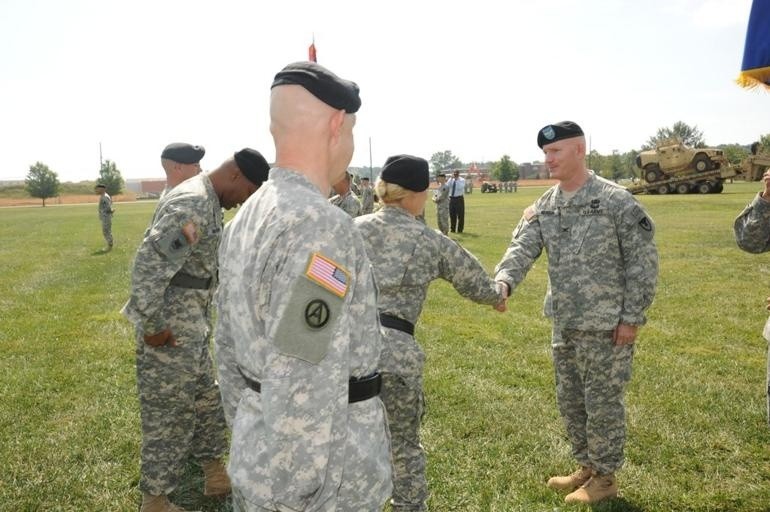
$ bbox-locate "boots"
[201,456,231,496]
[141,491,184,512]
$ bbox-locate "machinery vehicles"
[625,135,770,195]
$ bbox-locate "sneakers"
[565,474,617,505]
[546,466,593,491]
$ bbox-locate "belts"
[170,273,211,289]
[380,315,414,334]
[236,366,380,404]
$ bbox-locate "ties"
[451,182,456,196]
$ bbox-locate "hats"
[164,143,205,164]
[234,148,270,186]
[454,170,458,174]
[380,155,429,190]
[537,121,582,149]
[361,177,369,180]
[270,64,361,113]
[438,174,446,177]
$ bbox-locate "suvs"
[630,136,724,183]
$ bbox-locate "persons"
[733,166,770,426]
[349,155,506,512]
[117,148,270,511]
[216,62,392,512]
[144,144,206,237]
[94,184,116,253]
[492,121,660,503]
[327,166,522,235]
